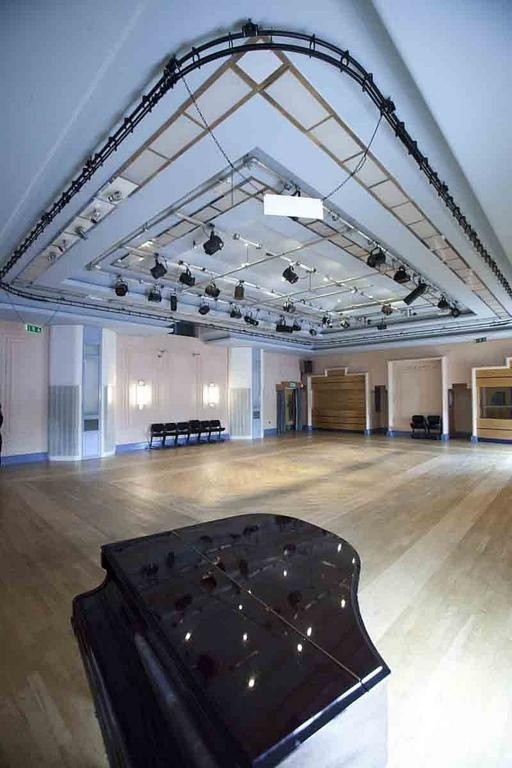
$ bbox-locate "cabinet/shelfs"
[312,370,365,431]
[476,359,512,441]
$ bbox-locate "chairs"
[426,416,442,440]
[410,416,426,438]
[150,420,225,448]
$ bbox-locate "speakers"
[174,322,196,337]
[301,359,312,374]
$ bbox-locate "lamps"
[245,316,259,326]
[114,280,128,297]
[234,285,245,300]
[284,304,295,312]
[309,329,317,335]
[230,310,242,319]
[208,383,216,408]
[322,317,332,329]
[170,293,178,312]
[148,288,162,304]
[282,268,298,284]
[150,264,167,279]
[137,380,145,410]
[394,271,411,284]
[292,324,301,331]
[203,236,223,256]
[178,273,196,286]
[204,285,220,298]
[381,306,393,316]
[437,300,449,308]
[451,308,458,318]
[366,253,386,269]
[340,321,350,329]
[287,190,302,222]
[403,282,428,306]
[376,324,387,330]
[276,324,293,333]
[198,306,210,315]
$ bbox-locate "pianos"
[70,513,391,766]
